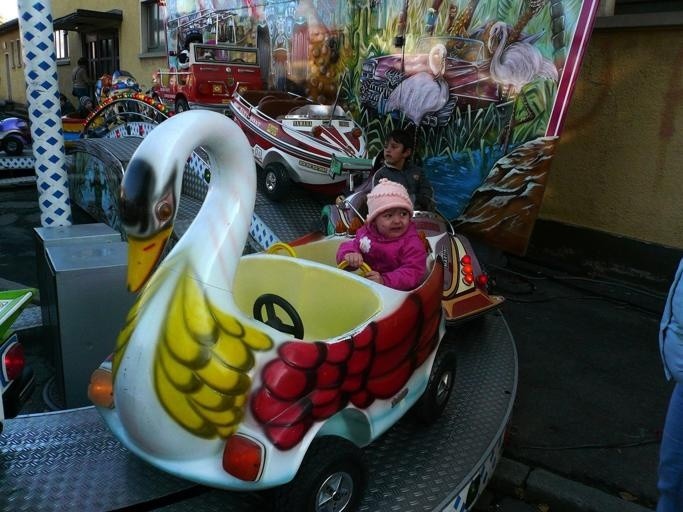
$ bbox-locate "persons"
[177,32,215,84]
[72,56,96,115]
[78,96,96,119]
[335,129,432,211]
[60,93,75,116]
[335,178,426,290]
[656,257,683,511]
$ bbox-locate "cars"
[358,20,536,127]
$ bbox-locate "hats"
[366,177,414,224]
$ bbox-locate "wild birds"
[381,42,450,182]
[488,21,559,158]
